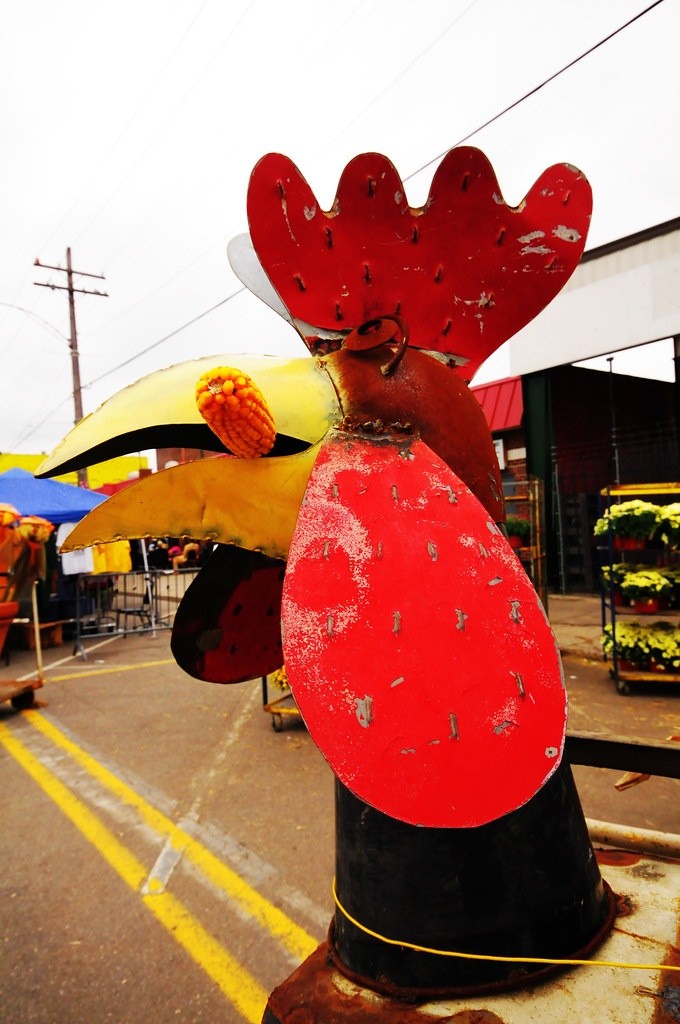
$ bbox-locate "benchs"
[24,621,65,649]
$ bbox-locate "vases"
[609,656,674,673]
[613,591,658,613]
[612,532,647,551]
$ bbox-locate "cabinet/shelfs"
[602,483,680,696]
[503,479,548,611]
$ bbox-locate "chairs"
[116,580,155,638]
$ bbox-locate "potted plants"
[506,515,529,549]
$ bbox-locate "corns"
[195,365,278,459]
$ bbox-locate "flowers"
[599,619,680,670]
[601,562,680,592]
[592,499,680,535]
